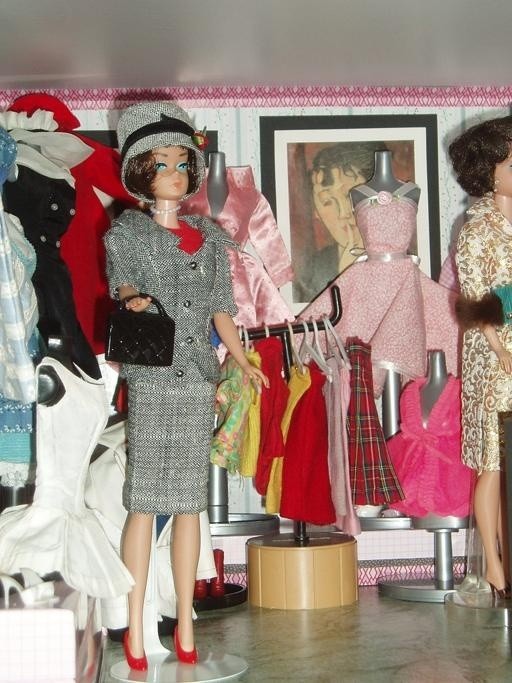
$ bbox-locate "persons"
[102,102,271,670]
[3,106,102,378]
[288,150,463,397]
[0,337,136,648]
[448,114,512,600]
[293,143,394,307]
[375,349,476,531]
[185,152,294,361]
